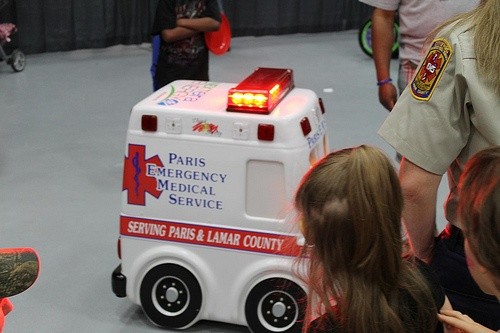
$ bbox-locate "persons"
[376,0,499,333]
[148,0,224,94]
[431,144,500,333]
[290,144,460,333]
[356,0,485,113]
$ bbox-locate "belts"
[443,220,464,243]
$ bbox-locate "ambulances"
[110,66,330,333]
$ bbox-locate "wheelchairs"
[358,12,399,59]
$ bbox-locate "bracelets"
[377,78,392,86]
